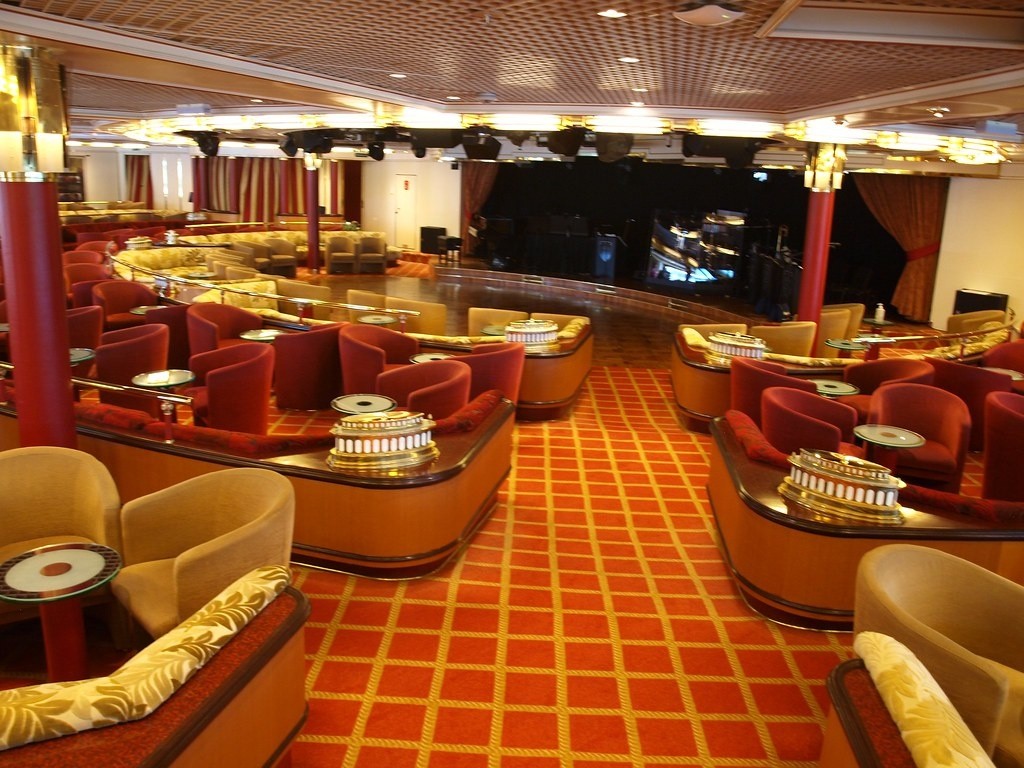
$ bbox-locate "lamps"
[751,0,1024,51]
[323,408,441,473]
[777,447,907,527]
[502,316,561,353]
[188,130,633,162]
[704,329,767,369]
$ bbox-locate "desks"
[357,313,396,325]
[807,378,860,398]
[853,424,926,477]
[408,351,456,365]
[128,304,169,315]
[131,369,196,389]
[0,541,125,684]
[857,331,896,360]
[330,393,398,416]
[69,346,96,368]
[861,318,894,334]
[824,336,866,360]
[238,328,288,341]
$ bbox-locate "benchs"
[106,240,595,423]
[0,385,519,583]
[176,221,403,268]
[669,307,1017,435]
[0,567,313,768]
[707,407,1024,632]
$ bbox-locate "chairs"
[0,223,1024,768]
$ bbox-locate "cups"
[164,230,178,243]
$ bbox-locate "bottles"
[874,303,886,322]
